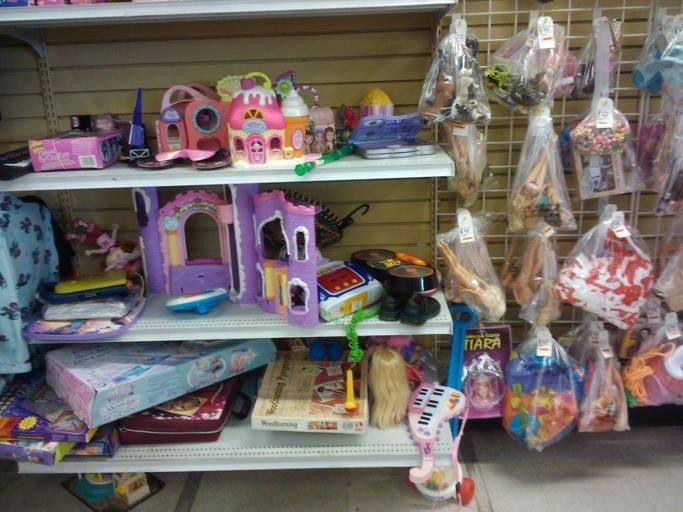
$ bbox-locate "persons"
[65,218,141,271]
[426,71,559,326]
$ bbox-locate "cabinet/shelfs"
[0,0,457,475]
